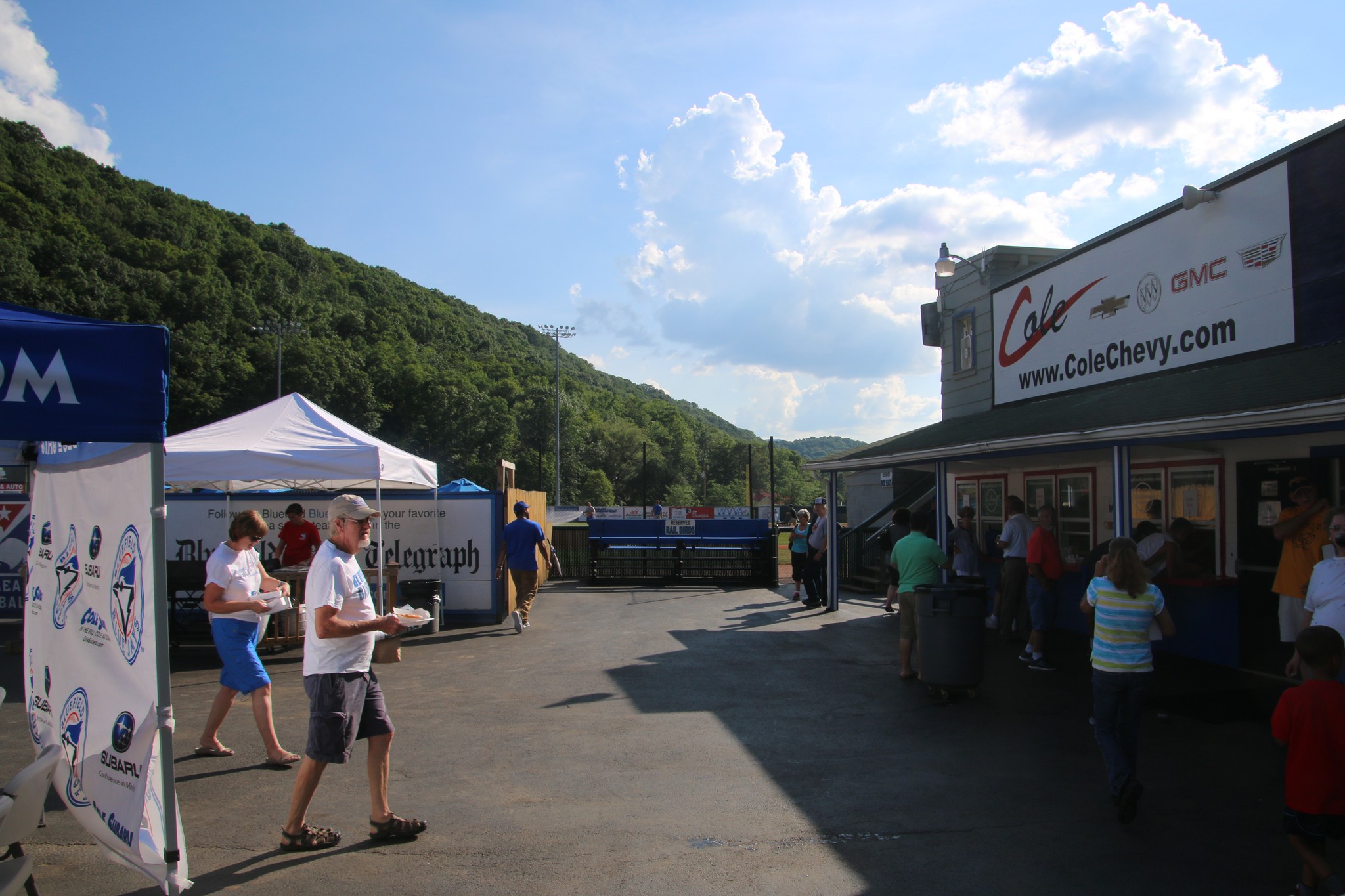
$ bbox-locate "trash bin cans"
[913,584,986,699]
[399,579,442,635]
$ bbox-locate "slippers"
[899,670,918,679]
[265,752,301,764]
[194,745,234,756]
[916,679,923,683]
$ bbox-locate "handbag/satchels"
[788,524,799,551]
[877,524,894,552]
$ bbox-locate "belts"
[1004,557,1027,561]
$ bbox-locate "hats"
[813,497,827,504]
[789,504,793,507]
[328,494,381,522]
[513,501,531,513]
[1288,475,1312,495]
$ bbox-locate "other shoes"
[885,607,894,613]
[523,622,530,628]
[1028,657,1058,670]
[1111,796,1120,804]
[1117,781,1144,824]
[512,611,523,633]
[1019,650,1032,662]
[792,591,823,609]
[984,615,1019,644]
[897,612,901,615]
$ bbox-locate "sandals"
[369,810,427,839]
[280,822,343,850]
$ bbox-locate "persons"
[196,494,555,852]
[652,500,663,519]
[775,477,1345,896]
[584,502,595,526]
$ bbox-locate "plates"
[393,614,435,626]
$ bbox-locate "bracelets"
[997,542,999,545]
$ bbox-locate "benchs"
[588,518,769,582]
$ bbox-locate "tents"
[0,302,493,896]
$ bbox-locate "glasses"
[340,516,375,525]
[1293,487,1313,499]
[249,535,263,542]
[961,514,971,518]
[1329,524,1345,531]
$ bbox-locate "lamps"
[1183,185,1217,210]
[935,243,984,286]
